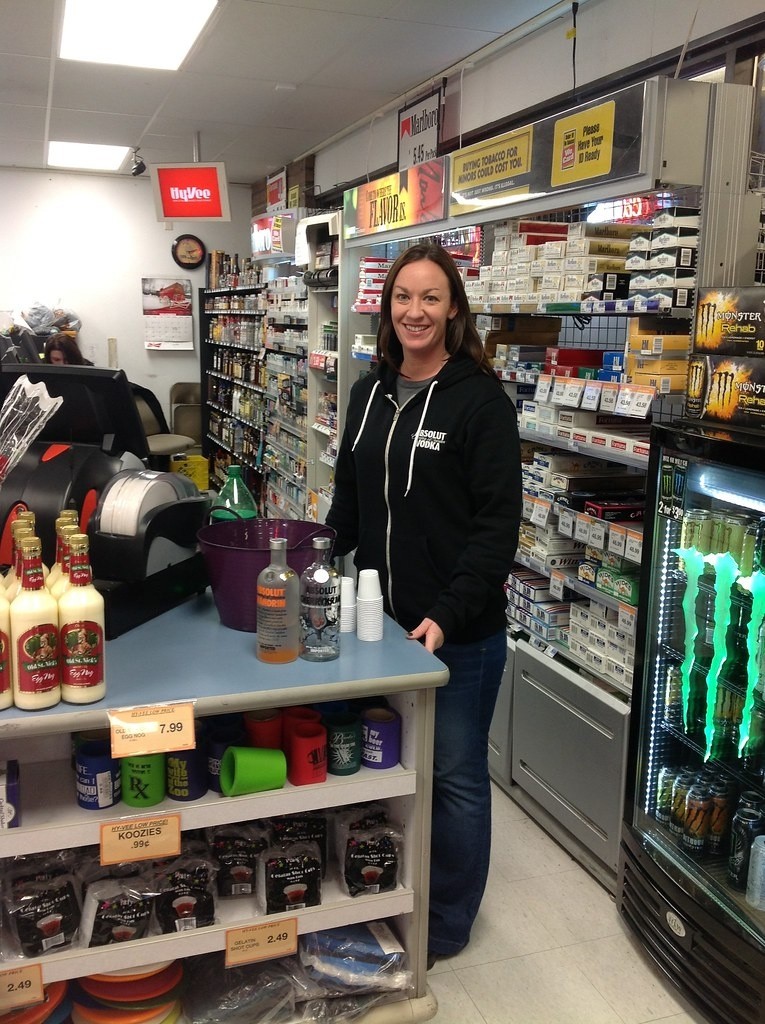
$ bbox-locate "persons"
[326,241,524,973]
[40,332,96,369]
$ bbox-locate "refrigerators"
[616,421,764,1024]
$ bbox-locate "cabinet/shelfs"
[352,303,692,704]
[198,282,308,520]
[294,211,373,525]
[0,586,451,1024]
[487,617,634,896]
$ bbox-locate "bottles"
[257,537,300,663]
[0,510,105,711]
[207,252,263,458]
[211,464,259,523]
[299,538,340,662]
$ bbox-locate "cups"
[340,569,384,643]
[70,695,401,810]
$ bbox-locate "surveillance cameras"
[131,161,146,176]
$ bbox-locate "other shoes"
[425,946,438,972]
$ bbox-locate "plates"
[0,952,183,1024]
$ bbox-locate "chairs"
[129,380,196,459]
[170,382,201,448]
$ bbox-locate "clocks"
[171,234,206,269]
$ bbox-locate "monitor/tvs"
[0,363,154,472]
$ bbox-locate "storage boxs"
[685,287,765,429]
[354,256,397,304]
[504,570,636,690]
[455,206,699,308]
[521,441,645,522]
[352,334,377,356]
[498,381,650,460]
[170,455,208,490]
[517,518,638,607]
[475,315,690,392]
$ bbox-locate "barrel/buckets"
[197,506,337,632]
